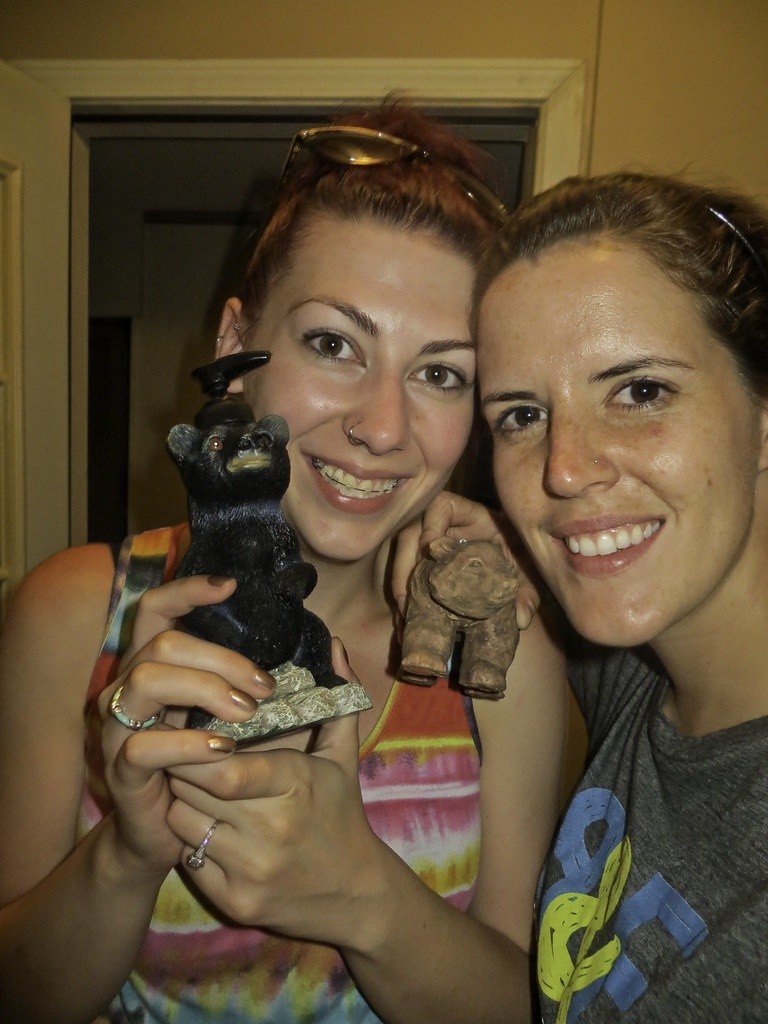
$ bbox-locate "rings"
[185,814,224,870]
[108,685,160,730]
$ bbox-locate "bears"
[163,413,348,729]
[396,536,520,701]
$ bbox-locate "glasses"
[255,126,509,277]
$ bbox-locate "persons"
[0,94,768,1024]
